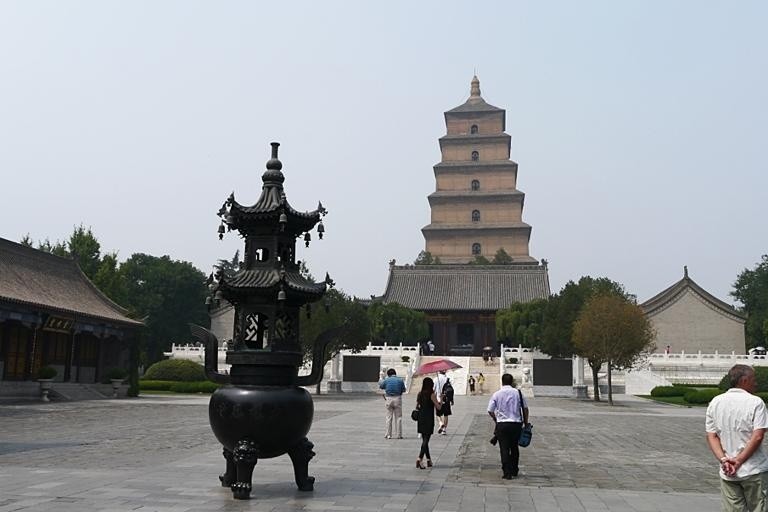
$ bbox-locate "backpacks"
[442,378,454,403]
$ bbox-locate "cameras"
[488,434,499,447]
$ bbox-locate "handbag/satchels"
[519,423,532,447]
[411,409,420,421]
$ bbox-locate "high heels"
[415,459,425,469]
[426,459,432,467]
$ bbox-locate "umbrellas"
[412,358,463,397]
[482,346,493,351]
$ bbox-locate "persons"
[432,369,454,434]
[468,375,476,395]
[665,343,670,353]
[483,349,489,366]
[428,340,435,354]
[487,372,529,480]
[487,350,494,365]
[704,364,768,512]
[476,373,486,394]
[222,338,226,346]
[380,368,406,440]
[415,377,444,469]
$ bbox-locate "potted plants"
[33,367,57,402]
[109,367,127,398]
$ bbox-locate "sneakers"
[502,471,518,479]
[438,424,446,435]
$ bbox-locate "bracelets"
[718,456,727,463]
